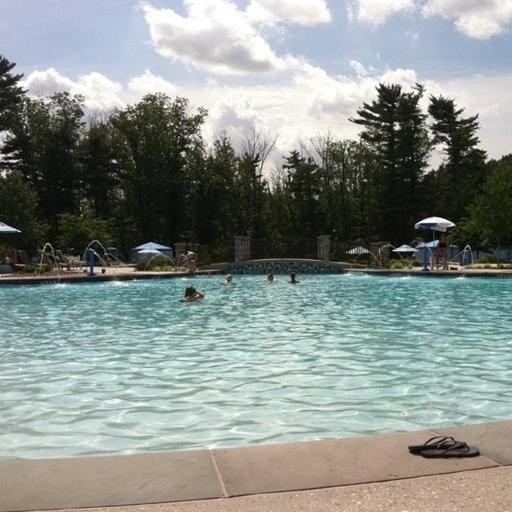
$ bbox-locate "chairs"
[38,248,87,273]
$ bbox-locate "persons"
[6,245,70,271]
[438,228,449,257]
[180,251,300,302]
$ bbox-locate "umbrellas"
[392,243,420,252]
[0,222,22,233]
[345,246,370,262]
[415,217,456,270]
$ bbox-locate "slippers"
[409,436,479,458]
